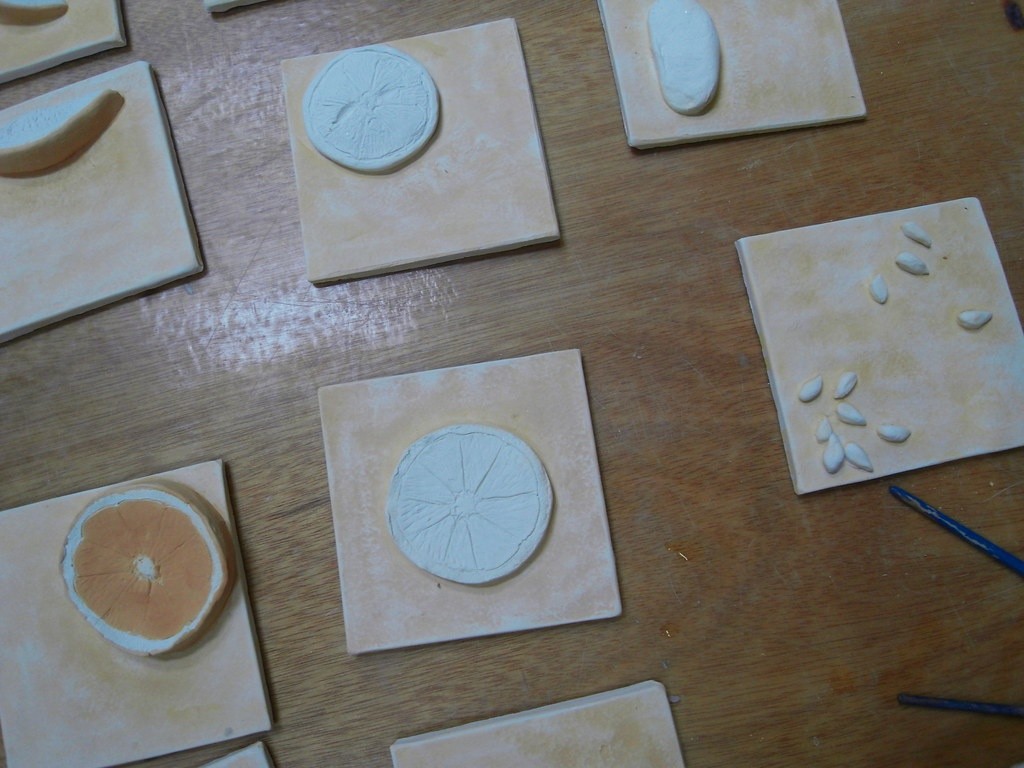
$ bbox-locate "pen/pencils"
[896,693,1024,719]
[887,484,1024,577]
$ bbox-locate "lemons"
[62,483,232,659]
